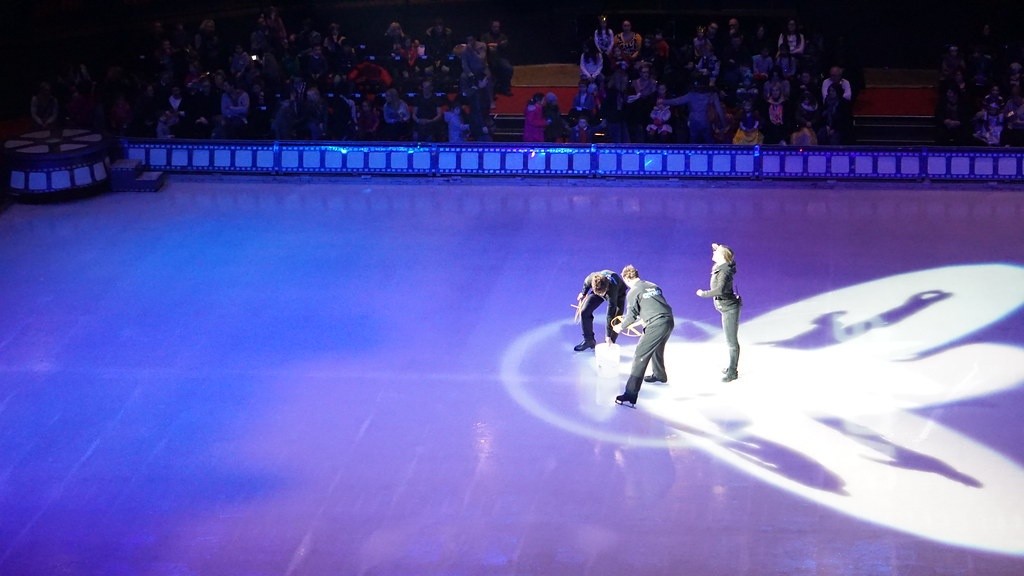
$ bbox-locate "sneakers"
[645,374,667,382]
[721,367,739,382]
[574,339,596,352]
[616,392,635,407]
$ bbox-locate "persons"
[696,241,744,383]
[566,6,1024,147]
[571,268,630,352]
[543,91,569,144]
[613,264,675,409]
[524,93,552,144]
[27,2,518,143]
